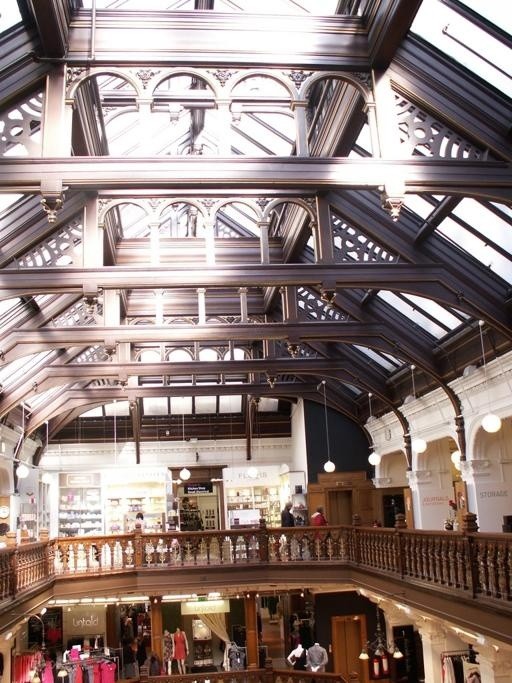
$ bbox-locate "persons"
[173,627,190,675]
[162,629,175,676]
[311,506,328,557]
[281,502,294,538]
[287,644,309,671]
[306,643,328,673]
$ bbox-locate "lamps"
[357,588,406,660]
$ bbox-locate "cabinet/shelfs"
[57,487,104,537]
[225,485,282,528]
